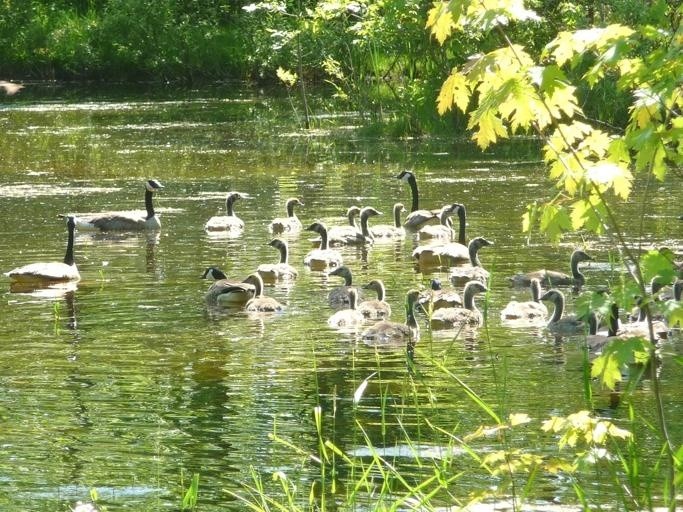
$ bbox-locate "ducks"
[241,272,284,314]
[200,266,257,309]
[305,169,682,349]
[258,238,298,284]
[270,196,305,238]
[206,191,245,234]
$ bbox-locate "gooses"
[87,179,165,232]
[85,232,160,278]
[3,216,81,283]
[11,284,77,331]
